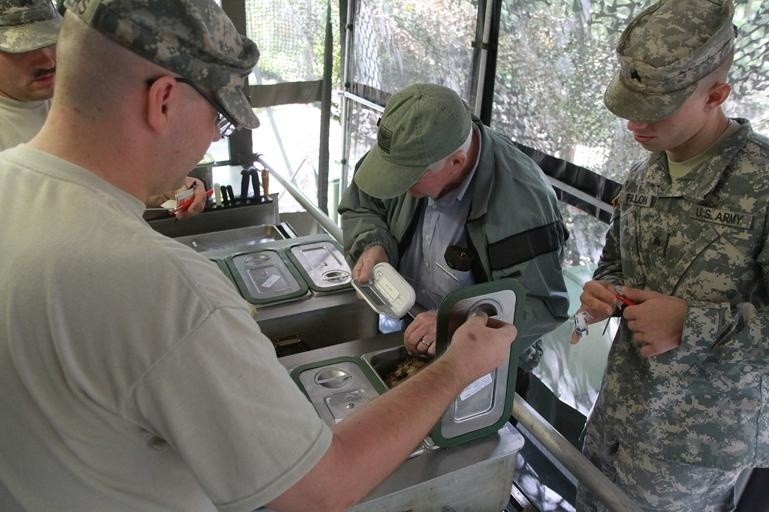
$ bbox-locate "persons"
[571,0,769,512]
[0,2,519,512]
[336,81,570,430]
[0,1,208,226]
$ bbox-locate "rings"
[421,339,430,347]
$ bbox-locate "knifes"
[214,168,272,208]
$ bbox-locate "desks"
[274,323,526,512]
[139,161,379,359]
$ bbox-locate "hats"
[60,0,263,129]
[1,0,66,56]
[353,81,470,201]
[603,0,737,123]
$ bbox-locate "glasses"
[141,76,239,138]
